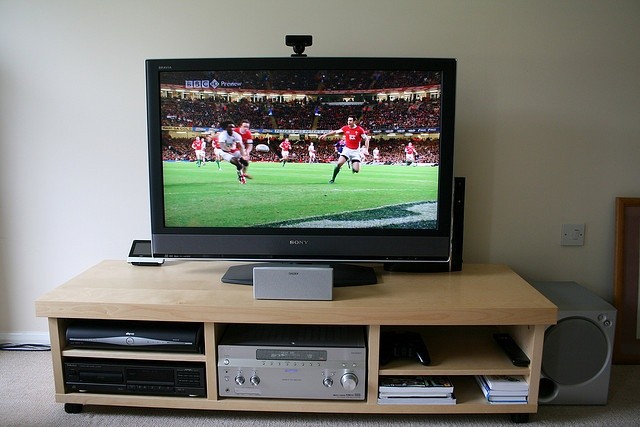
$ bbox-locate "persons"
[212,131,222,170]
[404,142,418,162]
[232,120,253,179]
[373,147,380,161]
[279,138,292,167]
[318,115,370,185]
[192,136,202,167]
[307,142,317,163]
[333,135,351,169]
[200,138,206,165]
[219,122,248,184]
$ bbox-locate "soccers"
[255,144,270,153]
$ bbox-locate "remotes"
[493,334,530,368]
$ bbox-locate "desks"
[35,259,559,423]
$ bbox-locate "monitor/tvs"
[142,57,457,288]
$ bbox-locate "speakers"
[528,281,618,407]
[252,266,334,302]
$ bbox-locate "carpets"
[1,340,639,427]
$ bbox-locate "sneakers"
[241,175,245,183]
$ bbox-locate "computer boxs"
[383,176,465,273]
